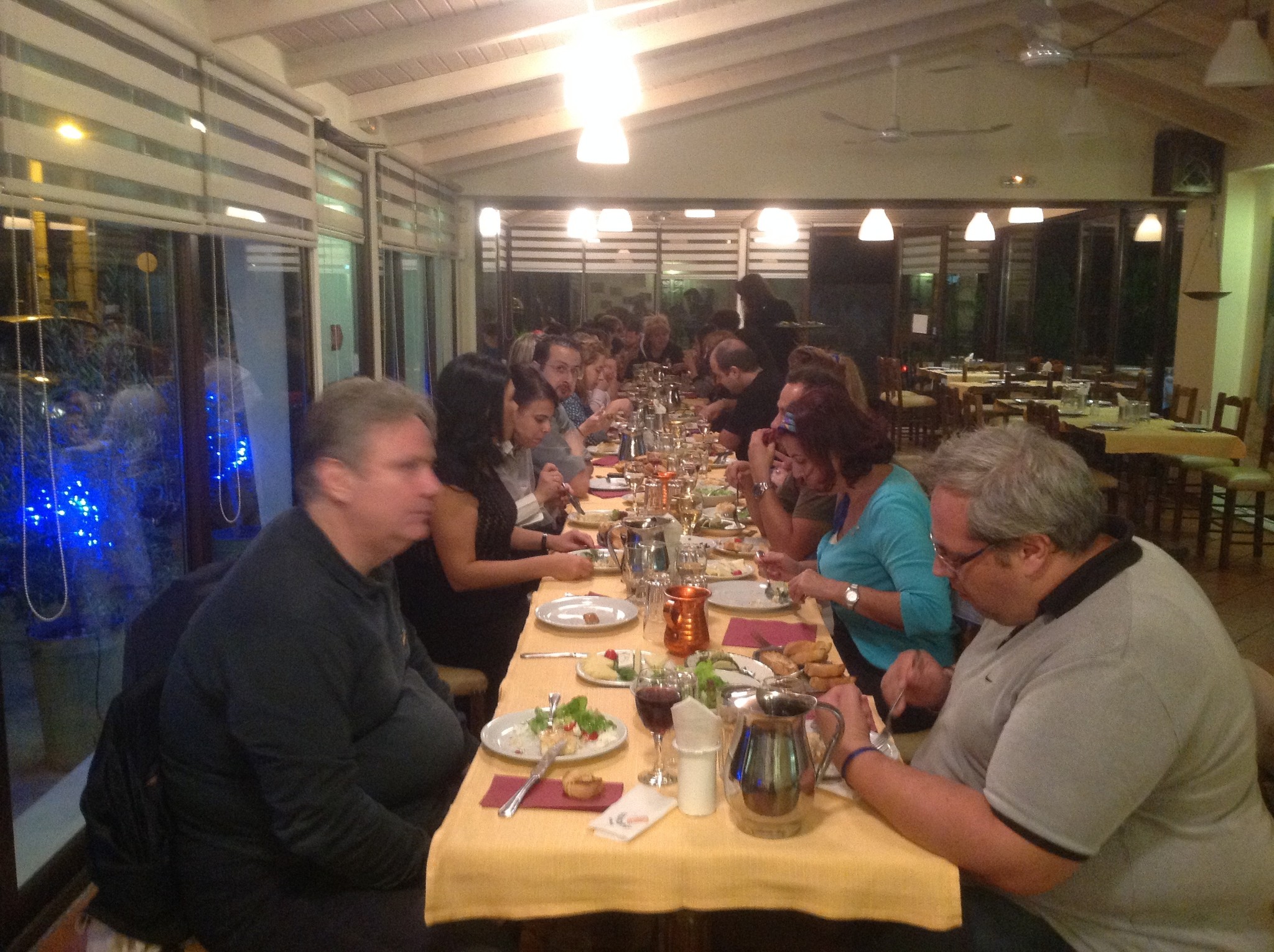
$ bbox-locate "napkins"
[653,399,666,413]
[671,696,721,746]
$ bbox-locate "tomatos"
[604,648,617,661]
[733,570,742,575]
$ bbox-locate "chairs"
[879,358,1274,576]
[80,661,214,952]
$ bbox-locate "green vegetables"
[611,509,628,521]
[696,508,749,534]
[695,487,734,496]
[582,548,604,562]
[529,695,617,736]
[613,659,639,681]
[668,659,734,709]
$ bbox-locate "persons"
[756,392,959,735]
[45,340,263,500]
[492,363,574,532]
[394,353,596,695]
[812,420,1274,952]
[508,273,869,560]
[157,375,522,952]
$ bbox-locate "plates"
[1011,398,1211,432]
[924,367,1023,385]
[480,707,628,761]
[480,443,900,781]
[775,321,826,328]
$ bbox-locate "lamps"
[598,208,635,232]
[576,121,630,163]
[1134,211,1162,242]
[1200,0,1274,89]
[1008,207,1044,223]
[963,210,996,241]
[858,209,894,241]
[1064,42,1109,141]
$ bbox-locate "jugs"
[605,515,675,593]
[656,382,683,412]
[652,365,668,383]
[717,683,846,841]
[617,423,648,462]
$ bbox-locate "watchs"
[843,583,860,611]
[752,481,776,499]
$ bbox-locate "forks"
[539,692,561,736]
[755,550,776,600]
[733,479,744,532]
[749,628,772,648]
[871,652,924,750]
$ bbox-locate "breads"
[715,501,736,514]
[761,639,858,689]
[614,463,632,472]
[724,541,754,553]
[606,551,622,568]
[610,529,623,549]
[562,767,604,799]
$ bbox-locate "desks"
[915,364,1006,397]
[998,398,1245,557]
[949,382,1066,432]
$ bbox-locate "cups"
[669,734,724,817]
[662,585,712,656]
[1059,365,1151,428]
[675,542,707,577]
[671,669,699,701]
[616,362,711,533]
[642,572,672,643]
[680,573,709,623]
[762,675,807,694]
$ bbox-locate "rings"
[554,476,558,482]
[775,469,780,475]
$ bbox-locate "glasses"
[930,531,1006,574]
[543,363,583,379]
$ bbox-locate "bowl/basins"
[680,534,717,556]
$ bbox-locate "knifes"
[520,652,588,657]
[497,736,567,818]
[560,480,586,516]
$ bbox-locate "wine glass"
[623,461,645,512]
[635,666,681,787]
[646,442,666,474]
[622,541,647,607]
[633,539,667,614]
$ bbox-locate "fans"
[931,0,1190,73]
[820,54,1016,144]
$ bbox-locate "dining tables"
[423,403,965,952]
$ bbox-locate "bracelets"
[541,531,549,555]
[723,397,730,410]
[576,426,586,438]
[840,745,879,782]
